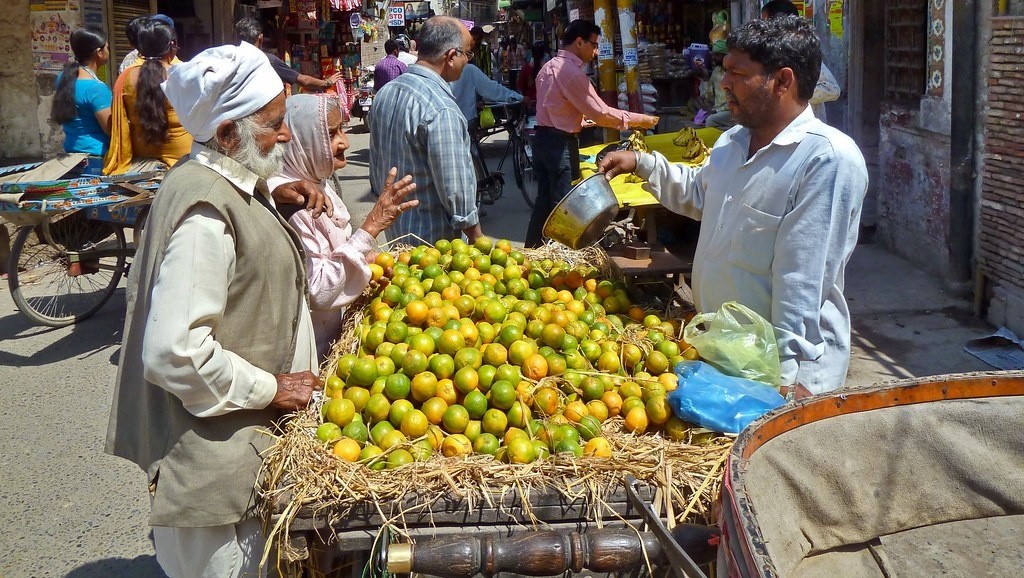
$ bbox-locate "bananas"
[624,129,652,183]
[679,96,714,120]
[673,125,711,167]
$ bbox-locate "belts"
[534,124,574,138]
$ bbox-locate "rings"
[398,205,403,213]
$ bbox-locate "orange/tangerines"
[314,236,716,472]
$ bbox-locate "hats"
[159,40,284,142]
[712,39,729,54]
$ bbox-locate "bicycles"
[469,99,536,220]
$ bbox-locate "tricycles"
[6,205,149,328]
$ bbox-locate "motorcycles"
[354,67,376,132]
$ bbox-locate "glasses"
[583,38,599,49]
[446,48,474,61]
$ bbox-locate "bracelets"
[630,149,639,175]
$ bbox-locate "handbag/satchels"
[479,106,495,129]
[683,302,785,391]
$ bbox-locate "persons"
[694,40,738,131]
[761,0,841,123]
[405,4,417,20]
[597,14,870,400]
[280,93,420,368]
[367,16,553,246]
[51,12,342,168]
[104,41,334,578]
[524,18,657,249]
